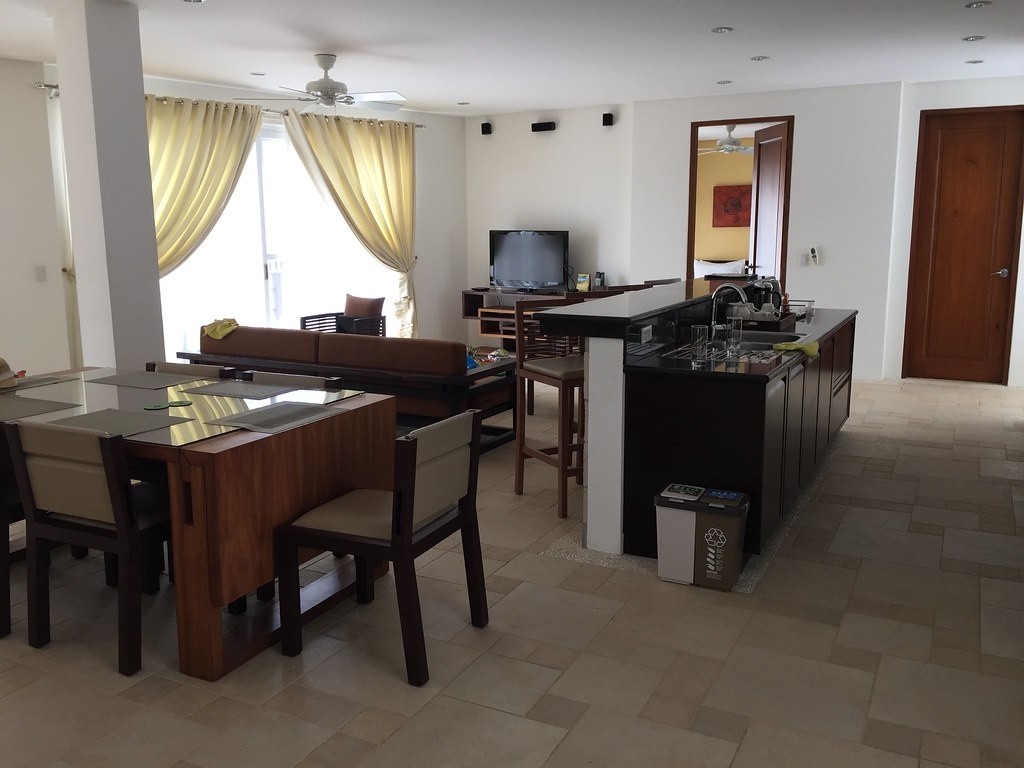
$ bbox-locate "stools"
[514,277,682,517]
[468,345,497,357]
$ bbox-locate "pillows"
[337,315,362,333]
[694,258,746,278]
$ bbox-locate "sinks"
[706,344,788,361]
[723,329,811,343]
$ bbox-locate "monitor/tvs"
[489,229,569,295]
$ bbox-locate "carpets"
[538,423,851,596]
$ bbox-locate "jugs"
[726,302,750,320]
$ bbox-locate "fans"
[698,125,754,156]
[233,54,407,115]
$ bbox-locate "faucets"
[710,282,748,344]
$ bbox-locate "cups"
[753,281,781,310]
[745,302,782,322]
[726,316,742,348]
[691,325,708,359]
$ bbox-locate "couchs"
[177,324,516,454]
[300,294,386,337]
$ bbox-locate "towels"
[202,317,240,340]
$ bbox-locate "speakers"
[603,113,614,126]
[481,123,492,135]
[532,121,555,132]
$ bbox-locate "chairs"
[271,409,490,685]
[145,360,234,582]
[0,420,119,639]
[4,419,247,675]
[243,371,345,600]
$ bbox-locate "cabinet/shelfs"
[760,316,857,555]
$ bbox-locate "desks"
[461,288,578,347]
[0,367,396,681]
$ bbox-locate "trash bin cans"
[694,489,750,592]
[653,482,706,586]
